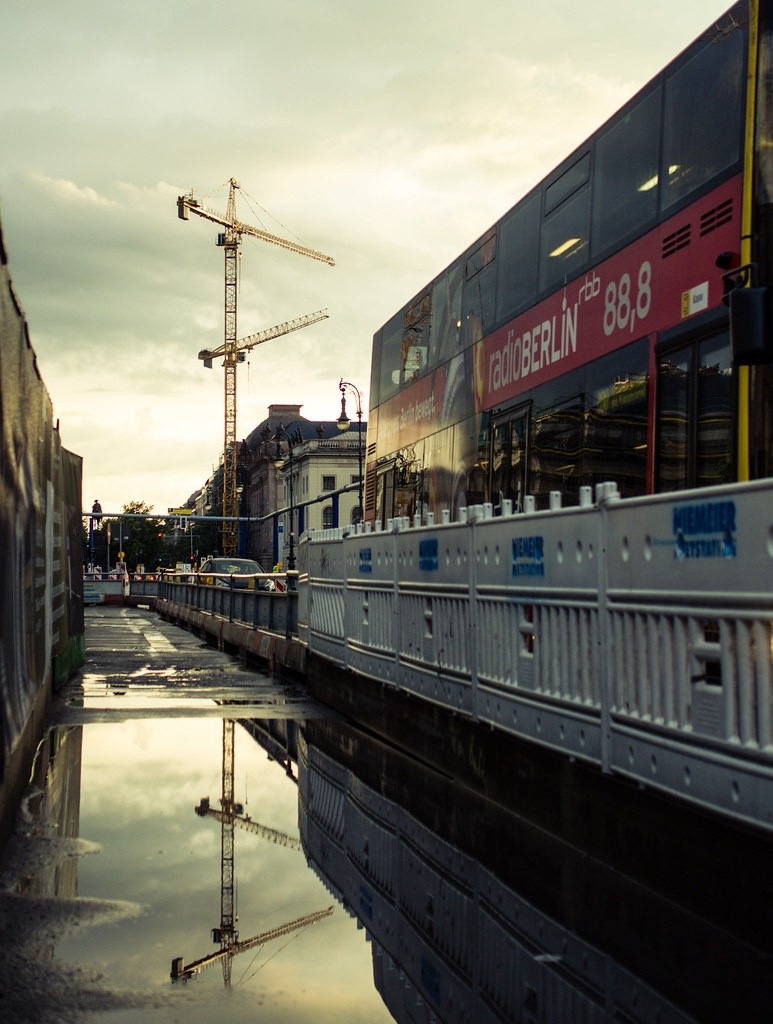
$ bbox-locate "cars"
[194,558,281,592]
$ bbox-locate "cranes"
[177,177,335,558]
[170,718,335,988]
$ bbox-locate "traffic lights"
[156,533,166,537]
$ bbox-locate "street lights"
[205,493,218,549]
[273,432,296,570]
[336,377,363,519]
[236,464,249,558]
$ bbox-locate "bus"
[362,0,773,533]
